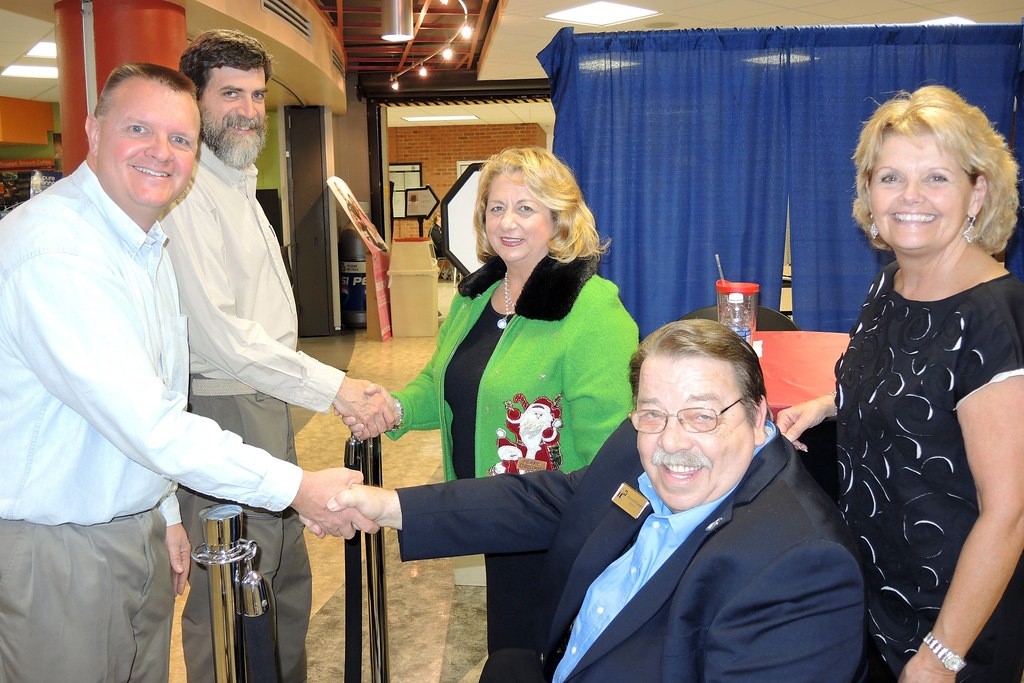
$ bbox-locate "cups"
[715,279,760,351]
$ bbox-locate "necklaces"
[497,272,533,329]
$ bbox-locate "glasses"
[627,397,746,434]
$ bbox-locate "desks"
[748,328,854,424]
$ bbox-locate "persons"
[0,29,399,683]
[344,148,641,683]
[299,317,866,683]
[773,84,1024,683]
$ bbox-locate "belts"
[192,377,257,396]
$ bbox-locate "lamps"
[380,0,414,42]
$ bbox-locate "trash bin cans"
[387,237,441,338]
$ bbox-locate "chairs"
[678,304,801,332]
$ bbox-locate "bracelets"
[393,397,403,432]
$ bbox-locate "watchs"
[923,632,967,673]
